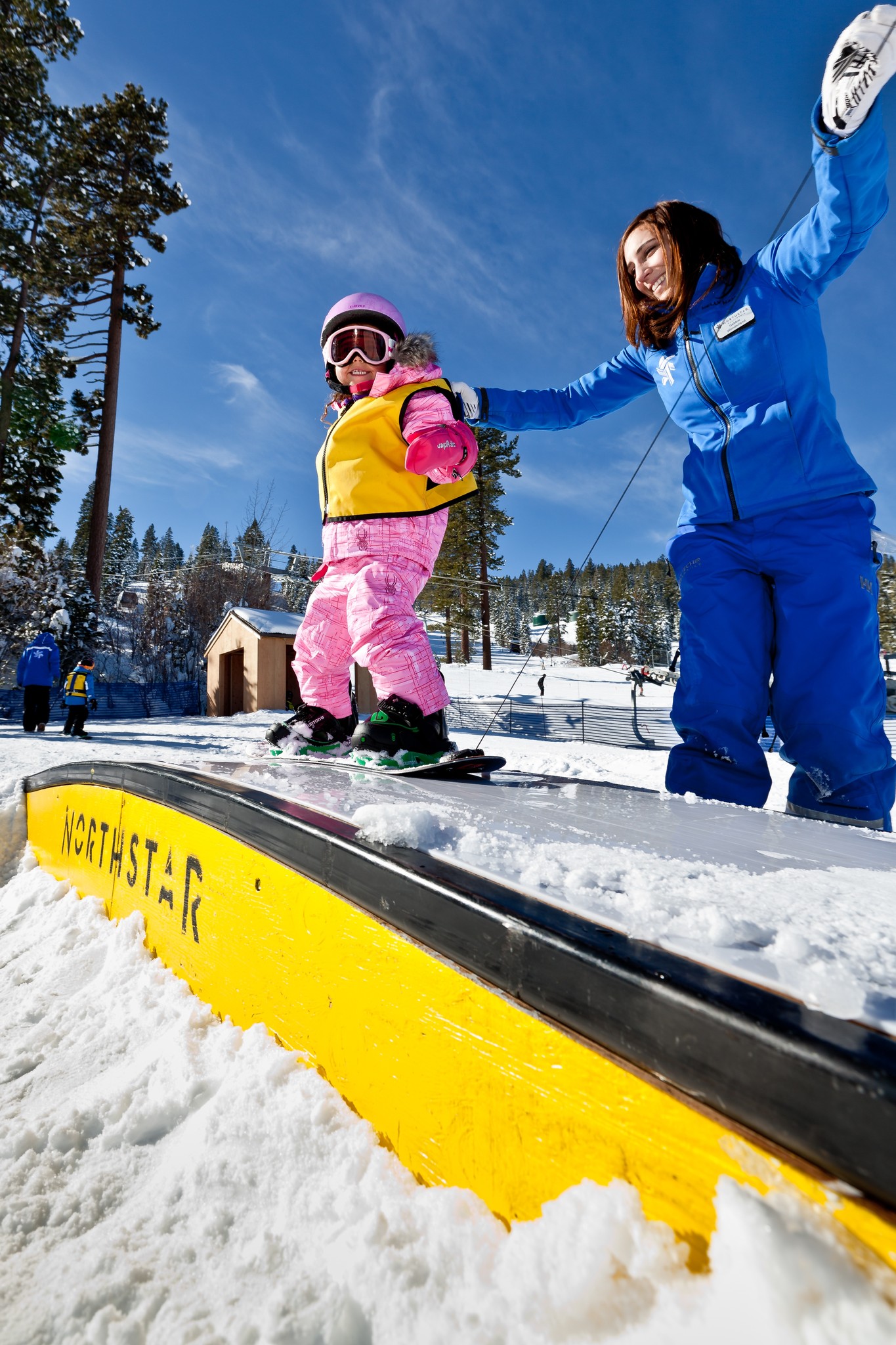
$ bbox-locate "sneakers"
[265,702,359,749]
[351,696,450,757]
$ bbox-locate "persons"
[60,659,98,736]
[266,292,478,769]
[16,628,60,732]
[540,657,546,670]
[538,674,546,696]
[451,2,896,840]
[638,684,643,696]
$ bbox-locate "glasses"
[322,325,402,368]
[77,659,95,669]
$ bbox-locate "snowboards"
[265,745,507,777]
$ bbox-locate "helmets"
[319,292,408,349]
[78,659,93,667]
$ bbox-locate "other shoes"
[63,729,70,734]
[37,723,46,732]
[71,730,88,736]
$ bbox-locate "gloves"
[60,699,68,709]
[819,2,896,141]
[90,698,98,711]
[449,380,479,421]
[404,424,467,476]
